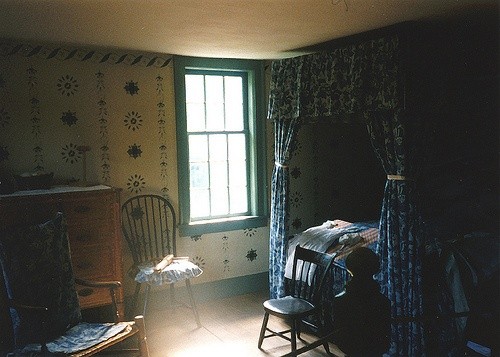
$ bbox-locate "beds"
[267,16,500,356]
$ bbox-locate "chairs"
[0,211,149,357]
[121,194,203,328]
[258,244,337,357]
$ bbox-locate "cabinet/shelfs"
[0,184,124,323]
